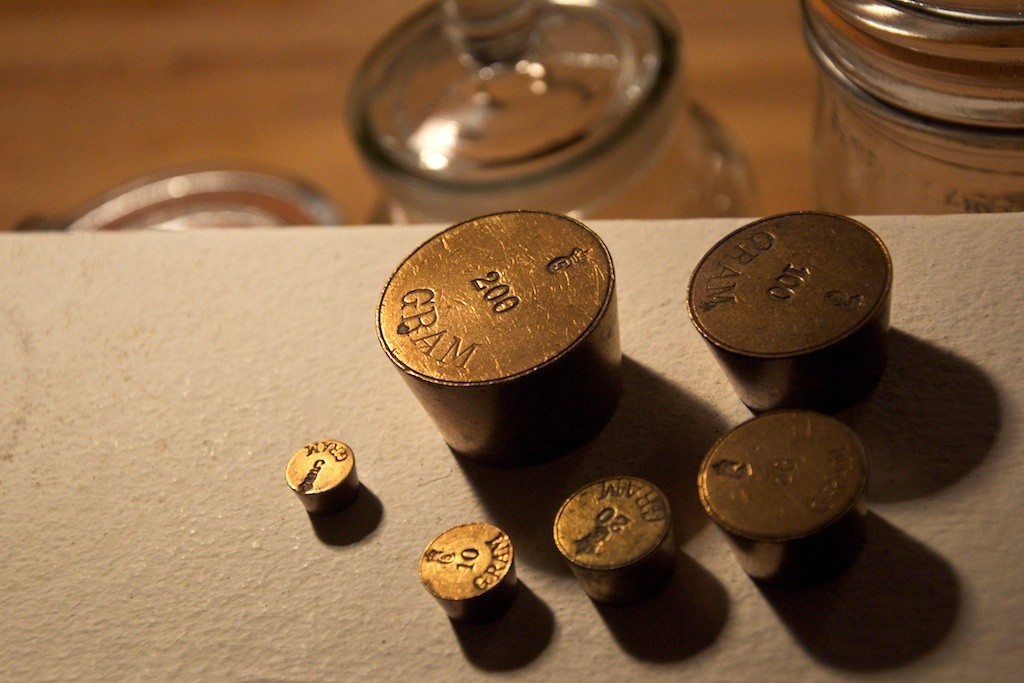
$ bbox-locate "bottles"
[58,162,336,231]
[796,0,1023,215]
[344,1,757,229]
